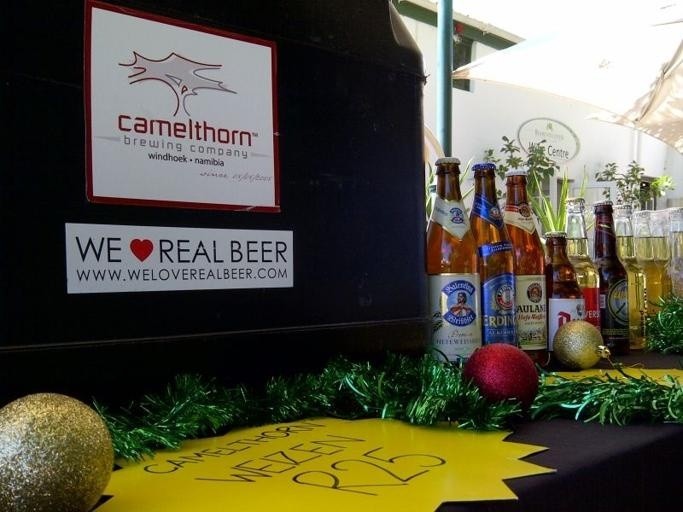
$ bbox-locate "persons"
[450,292,472,316]
[529,287,541,302]
[500,290,512,307]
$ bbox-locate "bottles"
[501,158,549,363]
[565,196,600,329]
[426,157,482,363]
[588,201,633,353]
[613,204,683,352]
[470,158,518,351]
[544,228,583,352]
